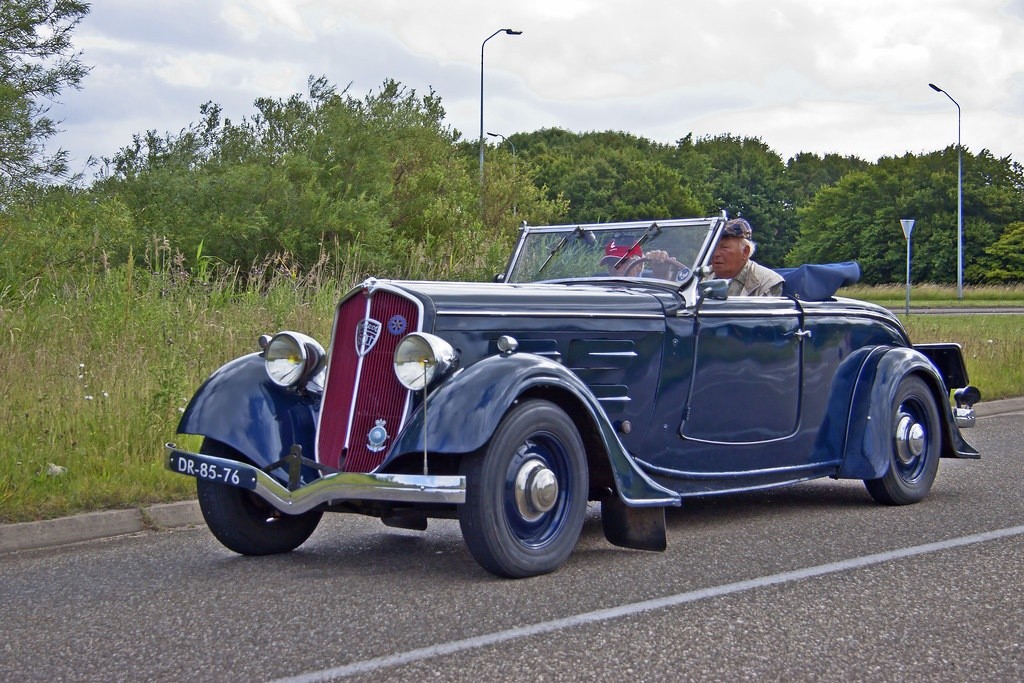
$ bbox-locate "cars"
[164,210,981,577]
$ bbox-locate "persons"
[645,218,786,297]
[599,237,645,277]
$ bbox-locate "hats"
[600,238,645,267]
[704,217,753,239]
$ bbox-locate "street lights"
[479,29,522,207]
[929,83,963,298]
[487,132,516,215]
